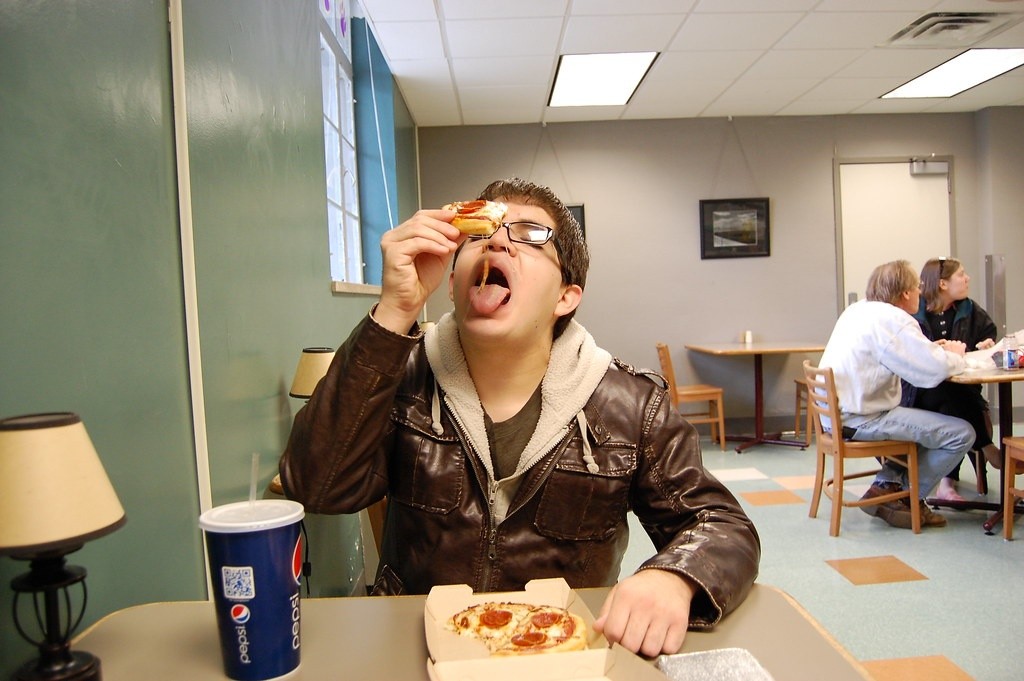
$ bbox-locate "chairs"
[1002,435,1024,542]
[655,343,724,452]
[793,378,820,448]
[803,359,921,537]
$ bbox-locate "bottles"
[1002,334,1020,371]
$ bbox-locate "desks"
[926,351,1024,534]
[68,580,871,681]
[687,342,826,454]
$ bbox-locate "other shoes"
[937,488,964,501]
[991,452,1024,476]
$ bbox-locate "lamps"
[1,413,126,681]
[288,345,337,399]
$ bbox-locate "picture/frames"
[698,195,772,259]
[565,204,588,242]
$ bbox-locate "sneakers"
[898,497,946,528]
[858,485,924,528]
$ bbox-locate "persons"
[814,260,976,529]
[875,256,1001,512]
[278,176,762,657]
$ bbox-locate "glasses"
[469,220,571,287]
[938,255,947,281]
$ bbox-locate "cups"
[199,499,306,681]
[739,330,752,343]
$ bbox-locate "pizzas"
[441,200,508,236]
[446,601,585,657]
